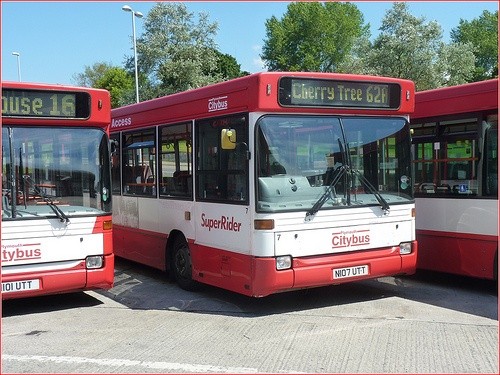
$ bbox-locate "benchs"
[123,166,143,182]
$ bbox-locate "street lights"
[11,51,20,80]
[121,4,146,103]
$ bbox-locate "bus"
[1,81,115,300]
[40,71,418,298]
[272,78,499,281]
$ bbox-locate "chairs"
[207,170,228,199]
[173,171,190,196]
